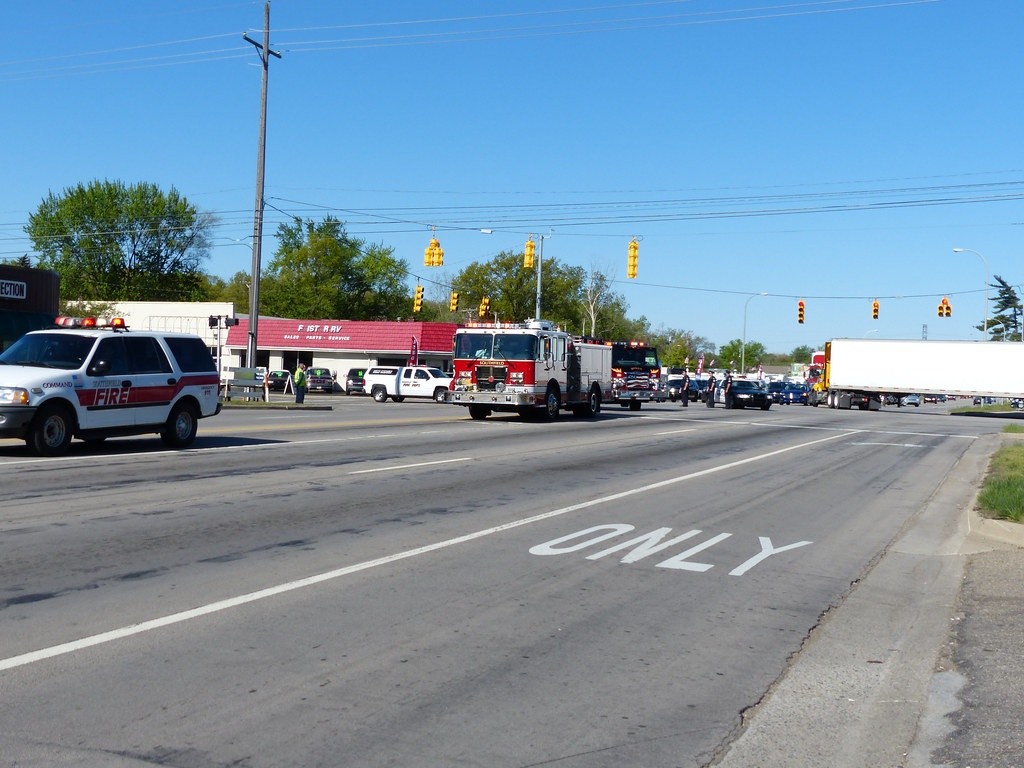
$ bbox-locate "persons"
[681,370,689,407]
[708,371,716,407]
[294,363,306,404]
[725,370,731,408]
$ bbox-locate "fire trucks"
[588,338,661,412]
[1,316,224,457]
[447,320,614,421]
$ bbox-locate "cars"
[660,377,811,411]
[901,393,996,408]
[265,366,368,397]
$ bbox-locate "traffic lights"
[478,296,491,317]
[413,285,425,312]
[937,299,952,318]
[798,301,804,323]
[450,293,459,312]
[872,301,879,320]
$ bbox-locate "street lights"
[862,329,879,338]
[741,292,769,375]
[986,317,1005,341]
[954,247,989,341]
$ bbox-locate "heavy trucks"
[805,337,1024,412]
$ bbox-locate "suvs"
[363,366,454,403]
